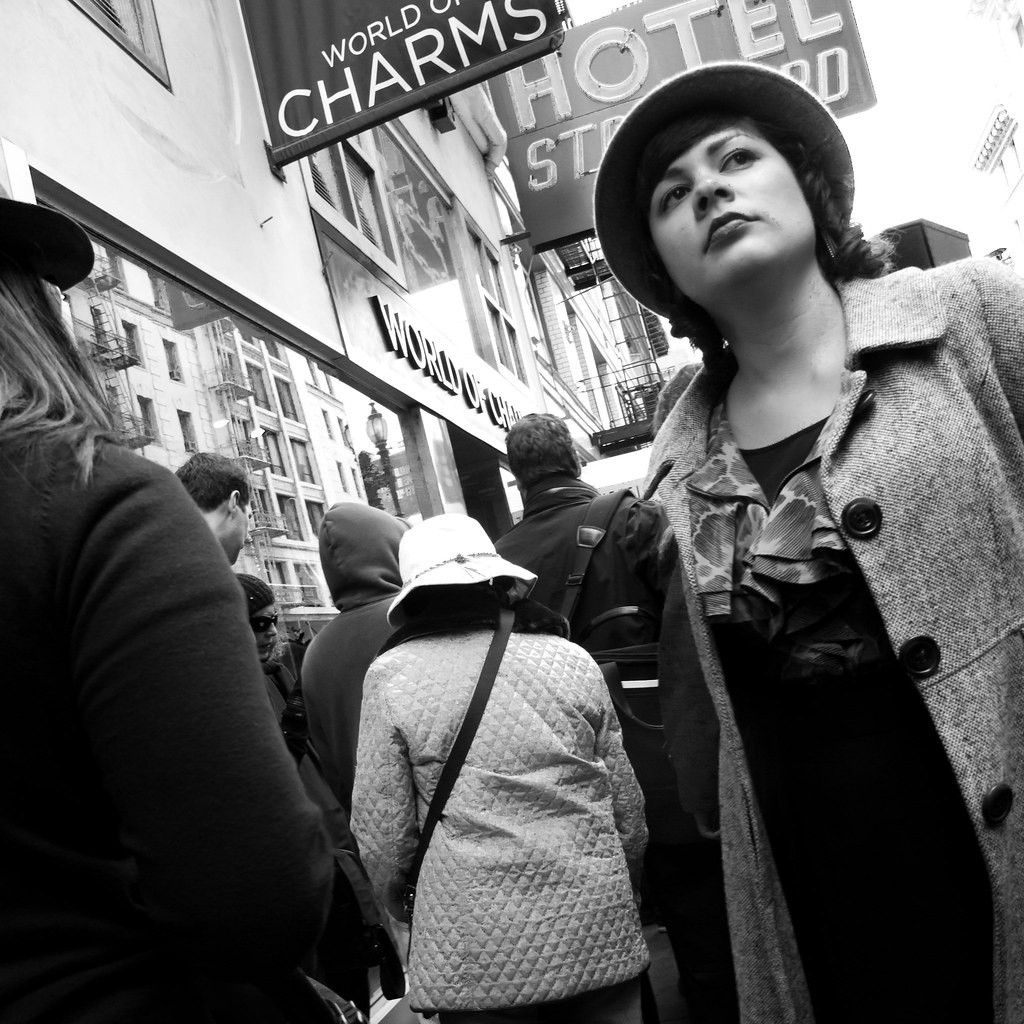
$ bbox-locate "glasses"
[249,611,278,632]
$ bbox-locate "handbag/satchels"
[547,490,683,803]
[315,846,405,1000]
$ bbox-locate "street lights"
[364,402,405,519]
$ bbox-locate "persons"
[0,197,336,1024]
[494,413,679,654]
[235,571,344,815]
[174,452,253,567]
[594,62,1024,1024]
[656,363,720,840]
[348,514,649,1024]
[301,498,411,817]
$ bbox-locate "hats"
[386,513,537,630]
[594,60,854,317]
[0,139,95,290]
[234,572,275,617]
